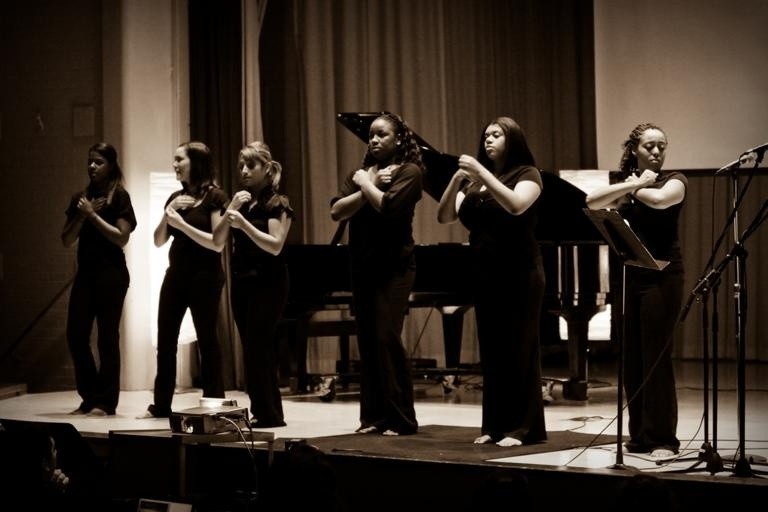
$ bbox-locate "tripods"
[656,162,768,478]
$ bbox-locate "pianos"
[279,111,612,404]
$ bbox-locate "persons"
[132,141,231,420]
[585,120,689,459]
[0,423,72,512]
[60,141,137,417]
[437,117,551,447]
[329,113,426,436]
[209,140,296,429]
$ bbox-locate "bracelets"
[87,210,94,218]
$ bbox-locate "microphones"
[718,148,757,176]
[745,142,768,154]
